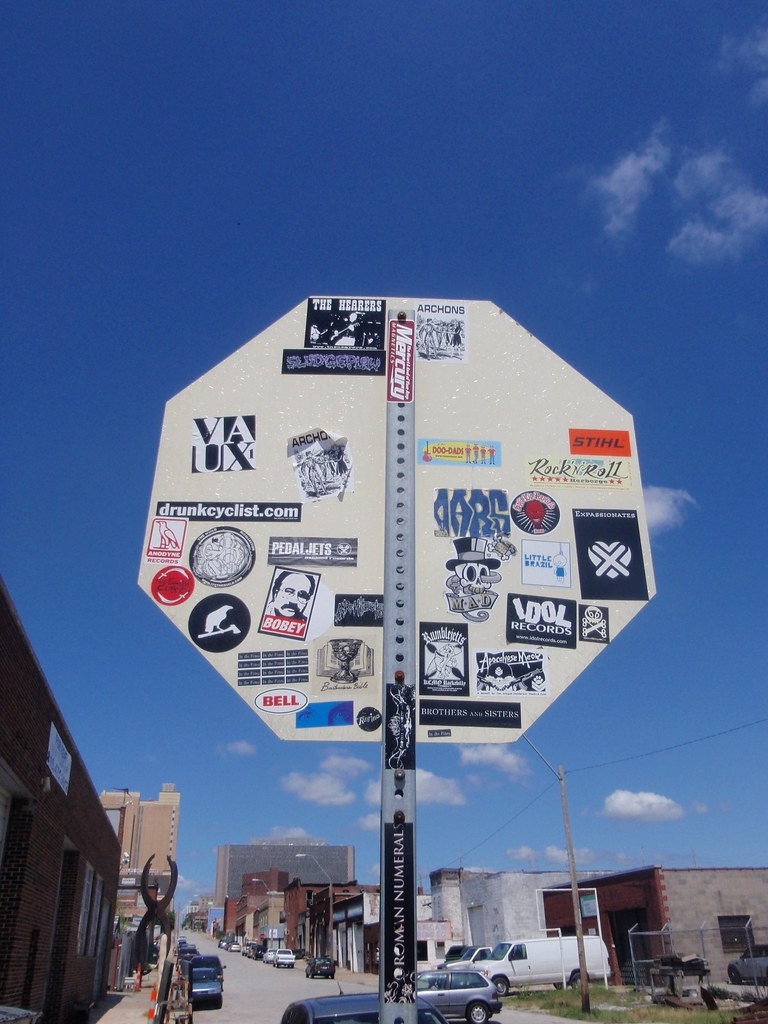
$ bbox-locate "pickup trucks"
[275,948,296,969]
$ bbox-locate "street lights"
[295,853,333,960]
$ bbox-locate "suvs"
[416,970,503,1024]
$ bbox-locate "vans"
[469,936,614,997]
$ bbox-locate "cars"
[281,992,449,1024]
[190,955,227,983]
[219,938,241,952]
[305,958,336,979]
[178,935,201,967]
[444,944,472,970]
[242,942,268,961]
[727,944,768,984]
[438,946,523,972]
[192,967,224,1008]
[263,949,279,965]
